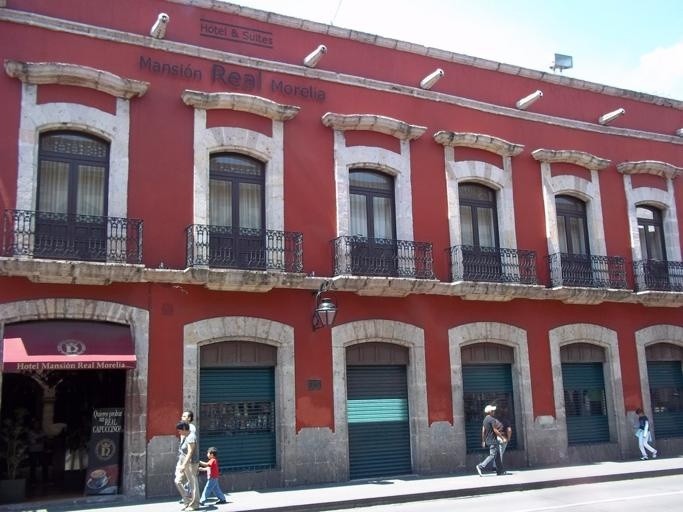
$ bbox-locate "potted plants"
[0,409,36,501]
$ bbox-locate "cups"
[90,470,106,487]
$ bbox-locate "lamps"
[550,54,572,72]
[312,290,338,330]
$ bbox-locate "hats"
[485,405,496,413]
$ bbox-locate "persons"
[173,410,226,511]
[476,400,513,477]
[634,408,658,461]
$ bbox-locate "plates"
[87,475,109,489]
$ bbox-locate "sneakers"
[179,500,203,512]
[476,465,481,476]
[216,500,226,504]
[641,451,657,460]
